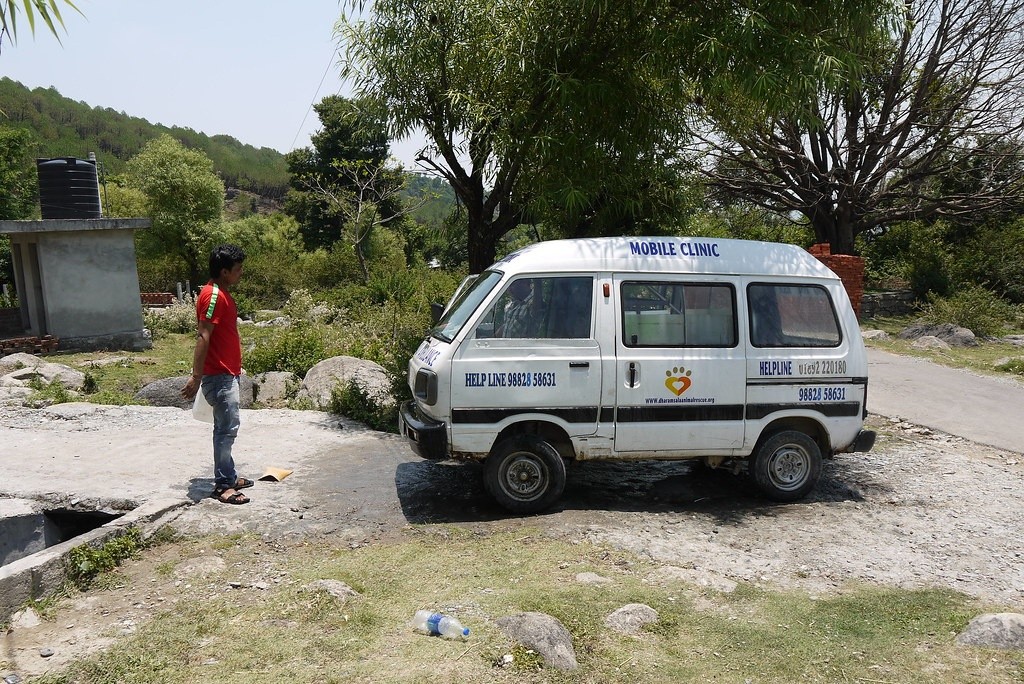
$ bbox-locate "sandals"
[211,487,250,504]
[235,478,254,488]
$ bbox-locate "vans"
[398,236,878,517]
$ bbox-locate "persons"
[178,247,254,504]
[492,280,550,339]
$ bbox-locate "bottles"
[412,609,470,638]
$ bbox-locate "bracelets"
[191,376,202,381]
